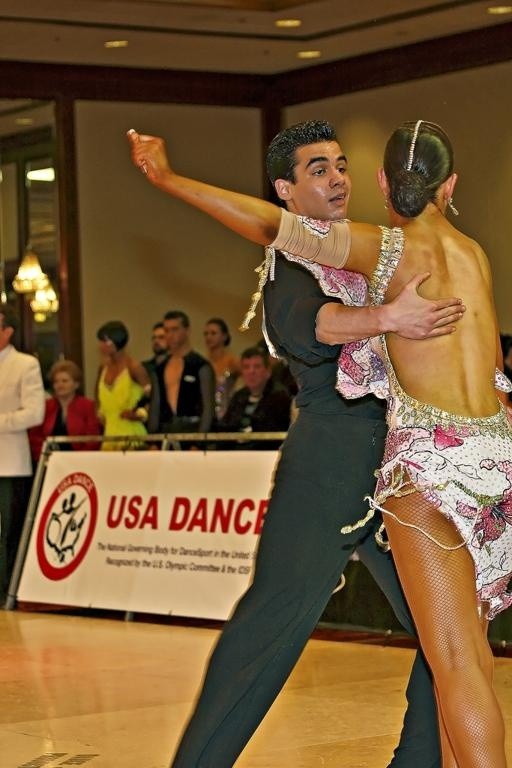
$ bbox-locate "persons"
[217,341,300,450]
[171,121,465,768]
[28,359,101,466]
[500,334,511,381]
[0,307,45,608]
[94,321,151,451]
[127,119,512,768]
[204,318,241,418]
[145,311,217,450]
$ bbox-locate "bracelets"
[137,406,148,421]
